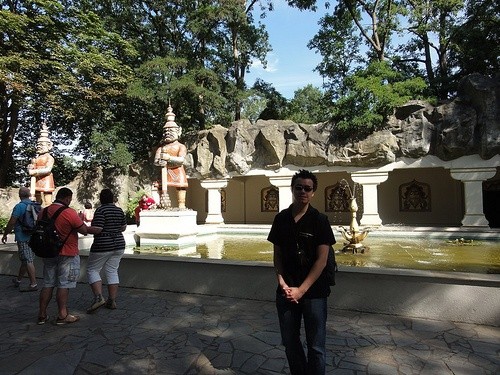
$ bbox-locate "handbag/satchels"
[321,246,338,296]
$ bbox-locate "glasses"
[292,185,314,192]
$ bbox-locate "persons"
[135,194,156,227]
[2,187,39,292]
[87,188,127,313]
[84,199,93,209]
[154,98,189,208]
[28,124,55,207]
[267,169,338,375]
[38,187,88,325]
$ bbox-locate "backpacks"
[19,200,44,231]
[28,206,71,257]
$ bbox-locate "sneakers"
[85,295,118,314]
[10,278,40,291]
[35,313,81,325]
